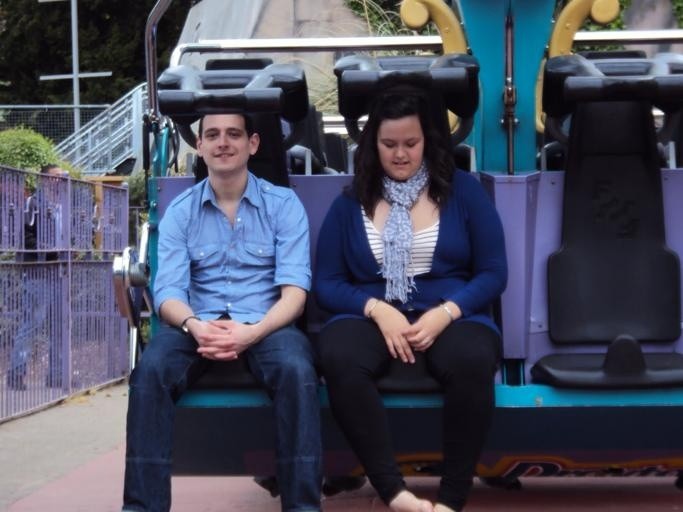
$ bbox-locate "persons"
[122,113,325,511]
[315,88,508,512]
[6,164,84,391]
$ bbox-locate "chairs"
[112,54,683,479]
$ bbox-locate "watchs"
[181,316,201,334]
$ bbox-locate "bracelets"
[440,304,454,322]
